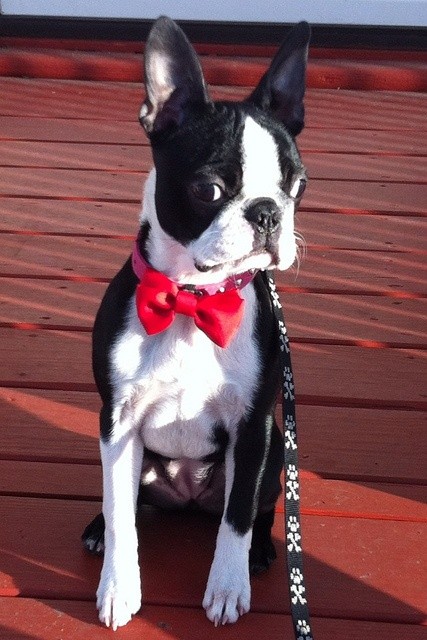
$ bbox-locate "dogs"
[82,14,312,633]
